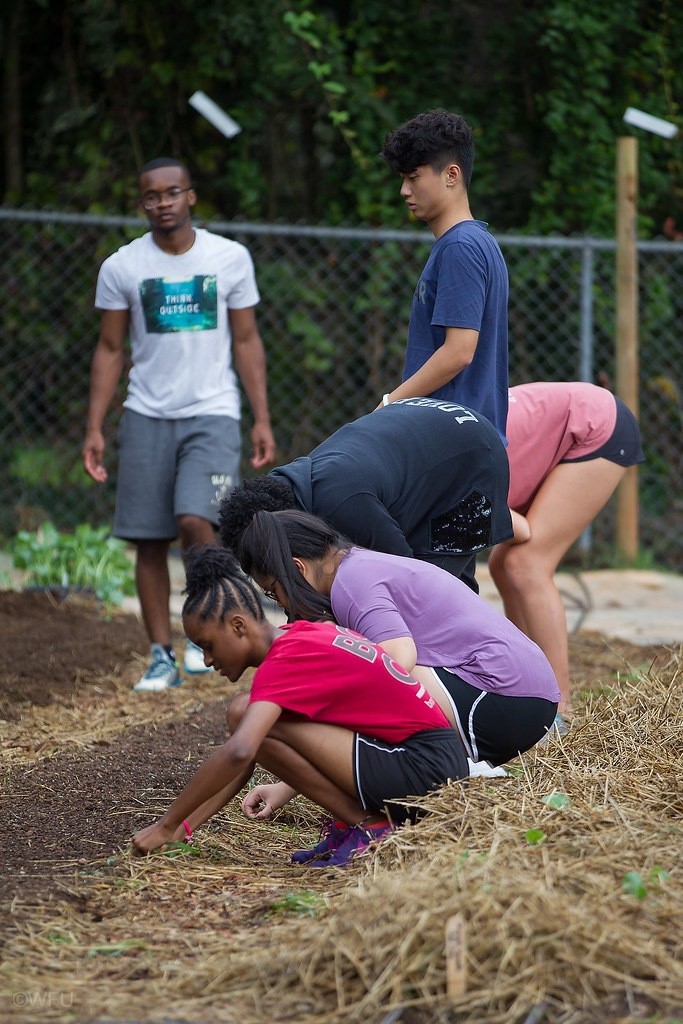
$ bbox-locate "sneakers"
[134,641,185,693]
[307,818,402,869]
[291,817,353,868]
[183,636,215,674]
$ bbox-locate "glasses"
[139,185,195,212]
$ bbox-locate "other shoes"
[536,714,576,747]
[466,757,508,778]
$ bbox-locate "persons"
[80,154,277,693]
[487,366,647,740]
[240,507,560,775]
[379,104,512,759]
[214,395,512,608]
[128,540,472,873]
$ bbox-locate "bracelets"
[182,819,194,842]
[382,393,389,407]
